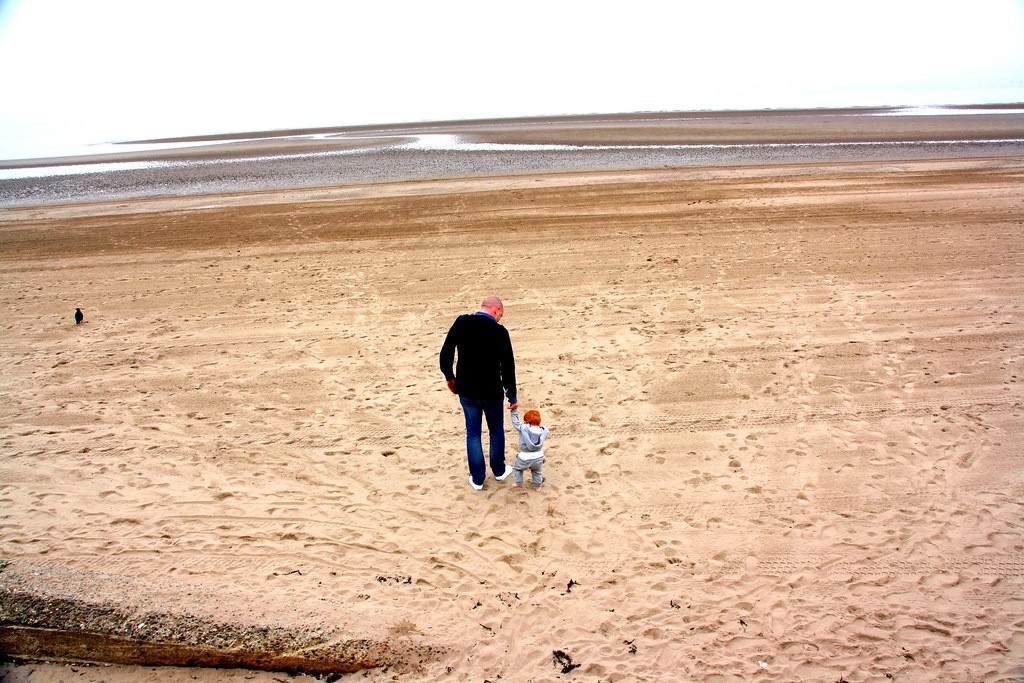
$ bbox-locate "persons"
[510,407,549,492]
[439,296,519,491]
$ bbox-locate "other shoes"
[496,465,512,480]
[533,485,542,491]
[469,476,483,490]
[512,484,523,488]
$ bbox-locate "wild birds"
[75,308,83,325]
